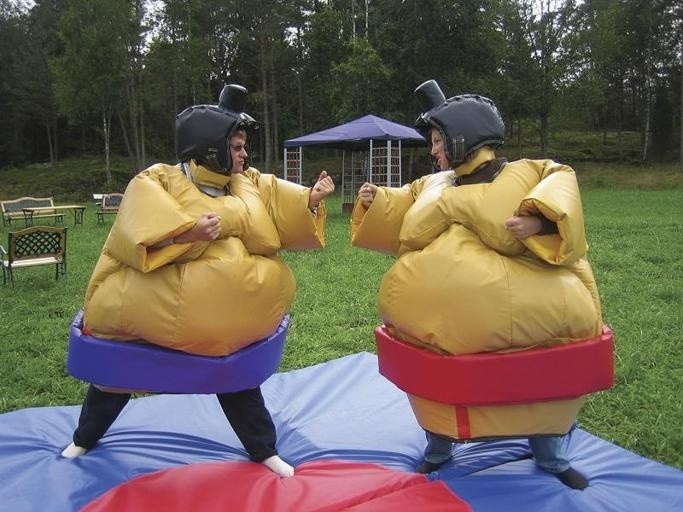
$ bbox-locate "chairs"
[96,193,124,223]
[0,225,68,287]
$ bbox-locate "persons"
[350,79,615,490]
[57,84,336,479]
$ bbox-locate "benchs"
[1,197,65,226]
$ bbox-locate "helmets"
[410,80,508,167]
[172,85,260,176]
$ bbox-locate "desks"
[22,206,87,228]
[93,193,107,221]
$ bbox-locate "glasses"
[230,142,247,153]
